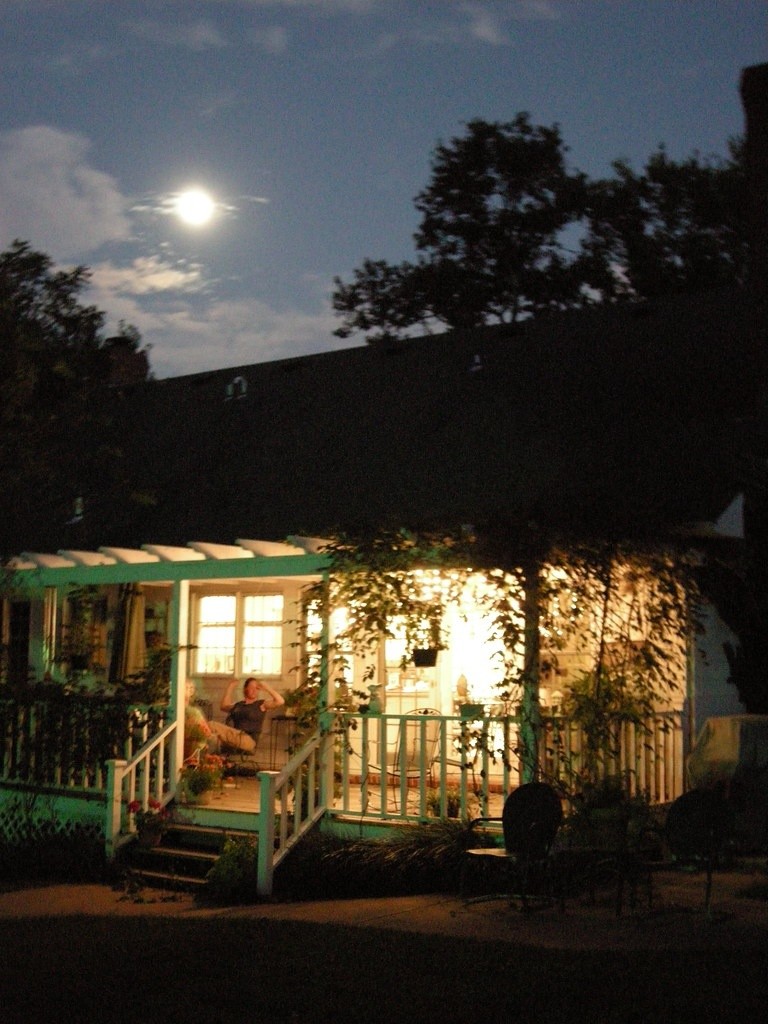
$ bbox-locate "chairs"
[444,783,552,919]
[423,719,499,814]
[622,789,725,913]
[365,707,445,818]
[212,719,268,787]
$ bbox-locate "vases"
[134,832,160,848]
[181,791,214,808]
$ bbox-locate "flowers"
[178,754,232,792]
[129,797,170,832]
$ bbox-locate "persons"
[184,678,286,755]
